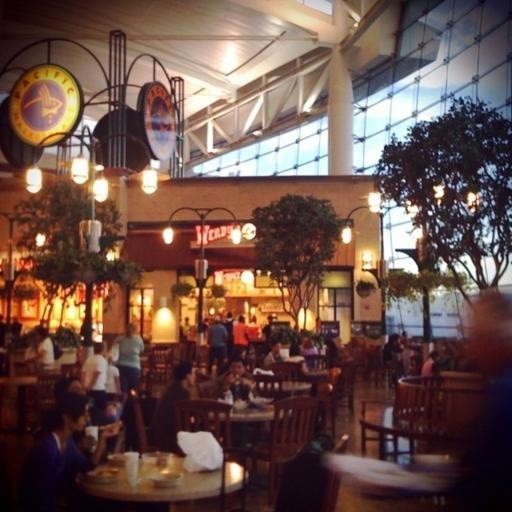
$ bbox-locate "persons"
[0,292,512,511]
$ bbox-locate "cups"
[124,452,139,484]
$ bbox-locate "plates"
[150,473,182,486]
[142,452,174,464]
[108,455,124,464]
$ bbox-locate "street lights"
[341,202,419,343]
[24,125,158,362]
[163,207,242,364]
[0,211,47,324]
[368,185,480,341]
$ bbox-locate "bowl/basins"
[87,469,122,483]
